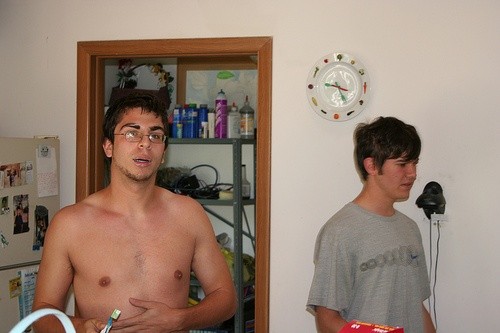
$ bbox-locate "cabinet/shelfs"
[162,136,256,333]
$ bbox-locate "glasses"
[112,130,166,144]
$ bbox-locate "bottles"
[171,89,227,140]
[227,102,240,139]
[241,164,251,200]
[239,96,254,138]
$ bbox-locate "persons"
[3,169,48,240]
[307,116,438,333]
[31,91,238,333]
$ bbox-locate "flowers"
[116,58,174,96]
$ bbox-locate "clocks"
[306,52,369,122]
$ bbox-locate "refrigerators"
[0,135,59,333]
